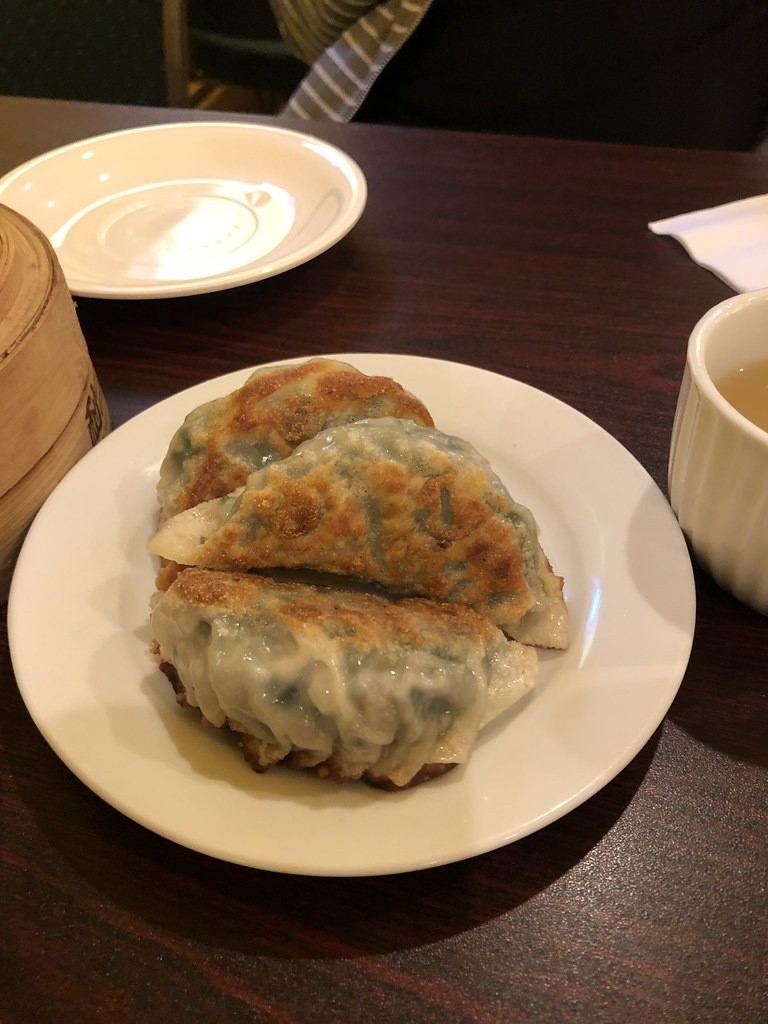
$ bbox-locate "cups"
[667,289,768,613]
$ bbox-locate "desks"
[0,95,768,1024]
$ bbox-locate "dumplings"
[143,358,569,790]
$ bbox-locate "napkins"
[648,195,768,294]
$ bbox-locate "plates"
[0,121,368,299]
[649,194,768,296]
[8,351,698,878]
